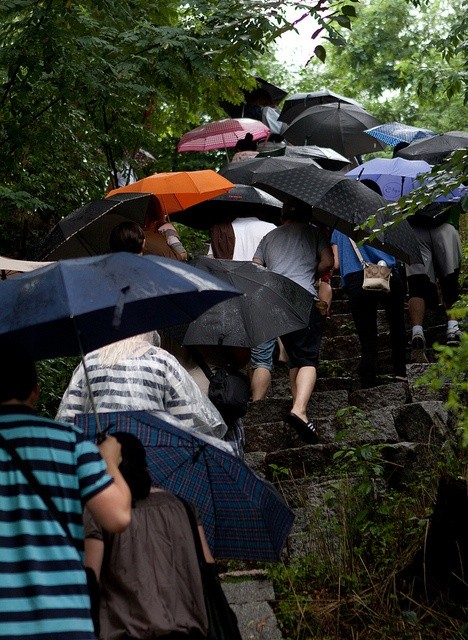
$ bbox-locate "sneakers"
[410,332,428,363]
[445,328,463,346]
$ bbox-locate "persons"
[184,346,246,457]
[0,348,133,639]
[110,222,146,256]
[404,204,464,350]
[250,195,337,445]
[331,179,413,383]
[85,429,241,640]
[143,194,189,261]
[206,205,279,401]
[55,330,195,431]
[107,158,136,191]
[230,133,259,164]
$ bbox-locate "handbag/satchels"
[208,364,250,417]
[362,260,393,292]
[200,558,242,640]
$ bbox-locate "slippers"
[284,413,317,445]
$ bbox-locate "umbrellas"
[162,253,315,348]
[216,156,323,184]
[280,99,388,158]
[391,129,467,164]
[250,164,425,266]
[344,157,467,203]
[177,118,270,165]
[37,194,162,262]
[0,250,246,444]
[254,136,353,170]
[277,88,364,127]
[0,256,57,280]
[103,169,236,222]
[217,74,289,123]
[68,407,297,563]
[362,119,437,146]
